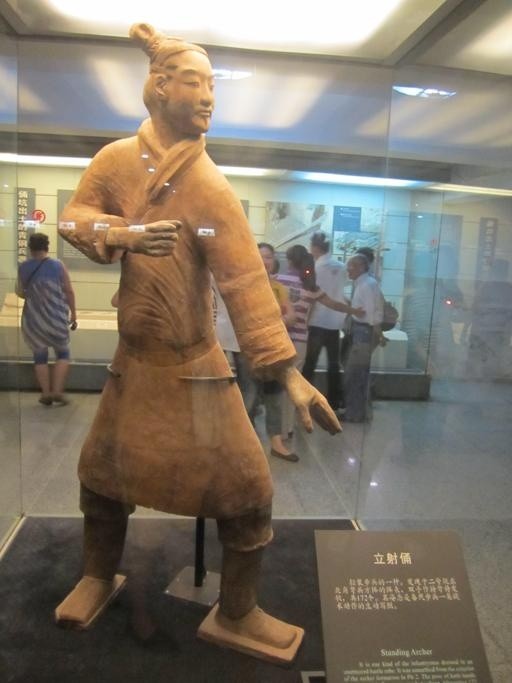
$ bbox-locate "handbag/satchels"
[383,299,398,330]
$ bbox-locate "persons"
[14,234,78,405]
[236,231,390,462]
[53,20,341,648]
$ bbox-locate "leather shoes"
[270,446,300,462]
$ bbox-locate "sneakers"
[39,394,71,405]
